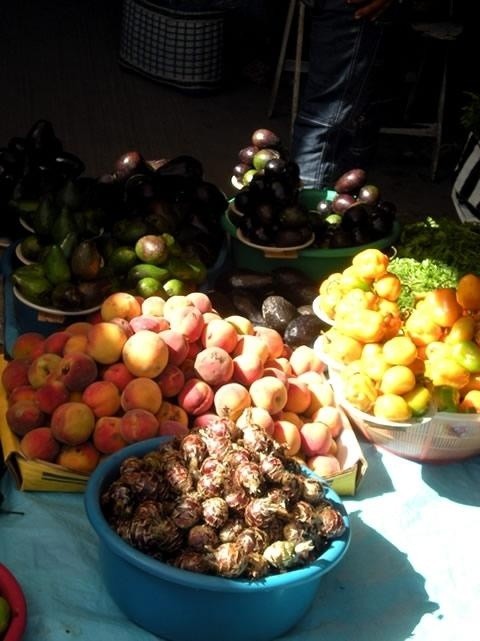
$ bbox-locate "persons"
[292,2,403,190]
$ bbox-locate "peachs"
[3,291,342,477]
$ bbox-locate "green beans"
[386,217,480,314]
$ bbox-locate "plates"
[11,215,102,316]
[225,198,318,252]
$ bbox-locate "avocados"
[0,119,396,348]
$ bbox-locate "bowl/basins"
[82,434,355,640]
[220,189,402,285]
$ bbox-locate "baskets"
[314,334,480,463]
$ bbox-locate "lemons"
[316,248,480,423]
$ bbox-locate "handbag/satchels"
[117,0,221,91]
